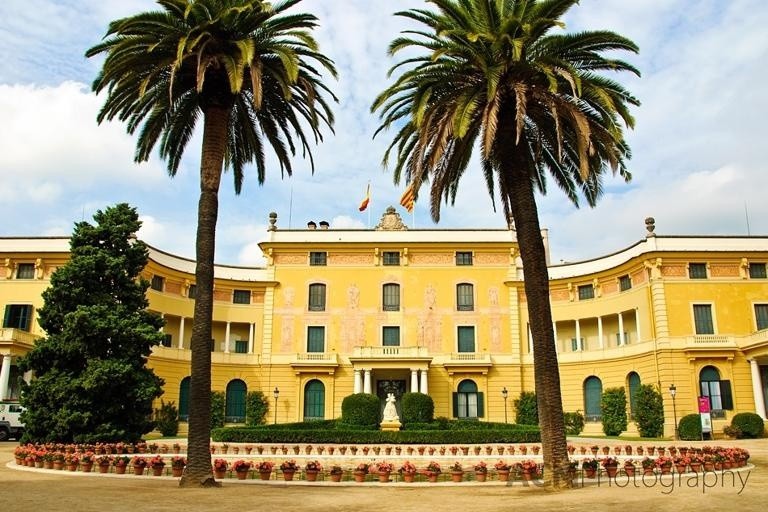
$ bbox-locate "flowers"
[14,442,749,473]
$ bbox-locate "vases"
[213,470,534,481]
[586,465,686,479]
[116,466,184,477]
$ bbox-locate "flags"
[359,183,370,211]
[400,181,415,213]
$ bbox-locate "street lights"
[669,383,680,440]
[501,386,508,424]
[273,385,279,423]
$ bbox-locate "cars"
[1,403,27,441]
